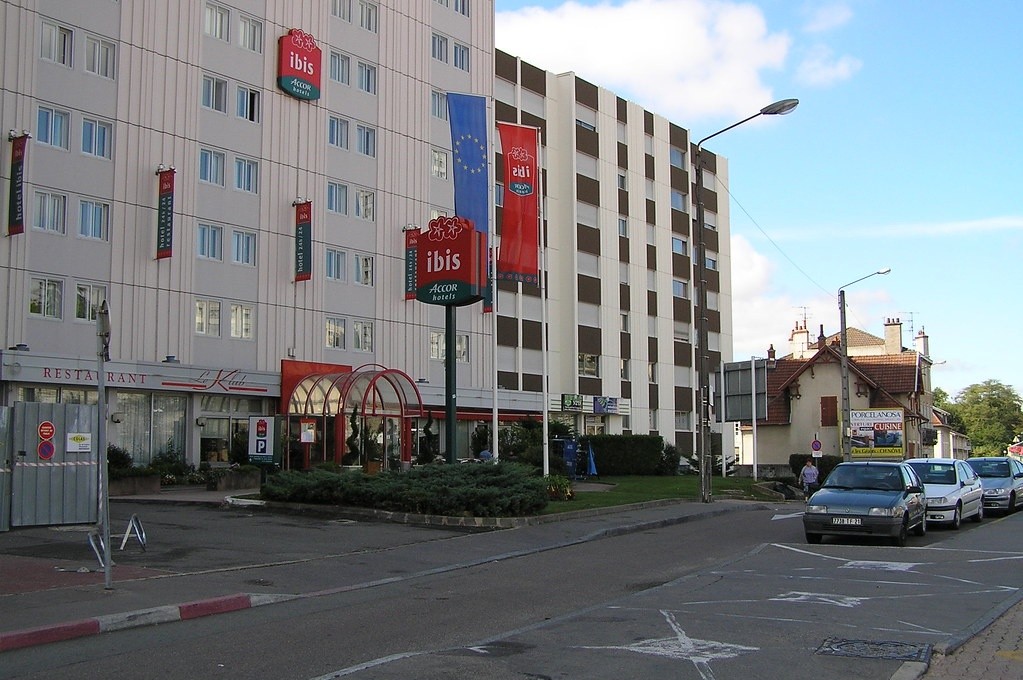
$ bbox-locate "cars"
[885,456,984,530]
[801,461,928,548]
[964,456,1023,515]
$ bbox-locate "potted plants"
[109,465,161,495]
[207,463,261,490]
[359,422,385,474]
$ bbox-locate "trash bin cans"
[260,462,278,493]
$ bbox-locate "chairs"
[944,469,955,483]
[884,476,900,488]
[997,464,1008,471]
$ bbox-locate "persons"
[798,458,818,501]
[481,446,492,461]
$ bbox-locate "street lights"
[837,267,892,462]
[695,98,802,505]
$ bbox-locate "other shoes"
[805,500,807,503]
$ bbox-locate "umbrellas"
[587,440,598,478]
